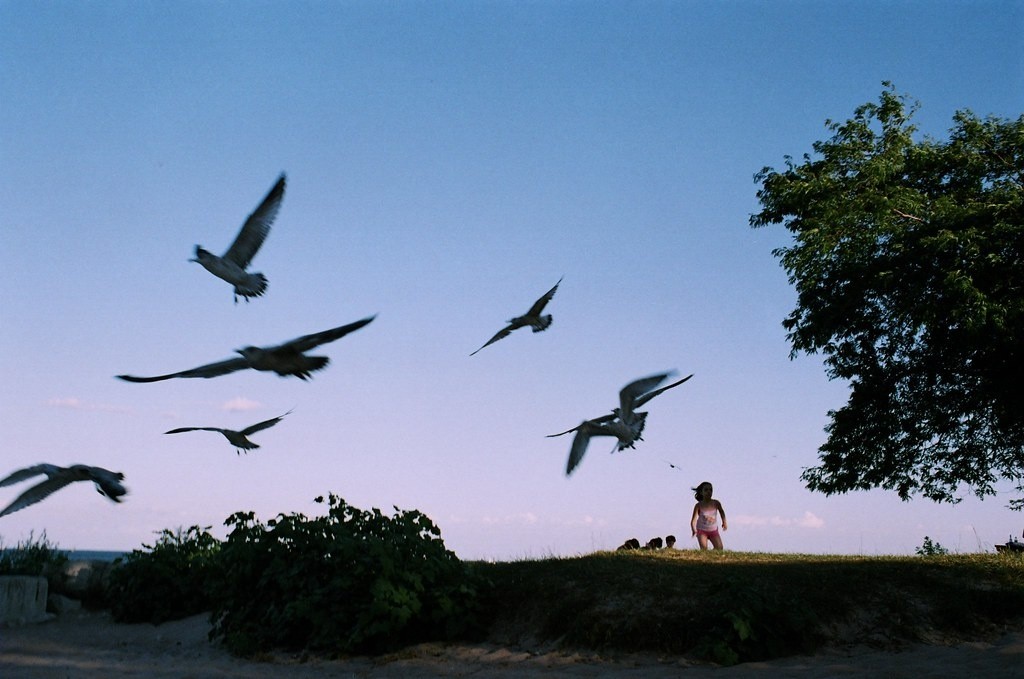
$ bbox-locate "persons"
[690,481,728,551]
[617,535,677,550]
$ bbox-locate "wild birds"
[113,313,377,384]
[469,275,566,357]
[544,370,695,478]
[163,406,293,456]
[0,464,127,517]
[187,170,288,304]
